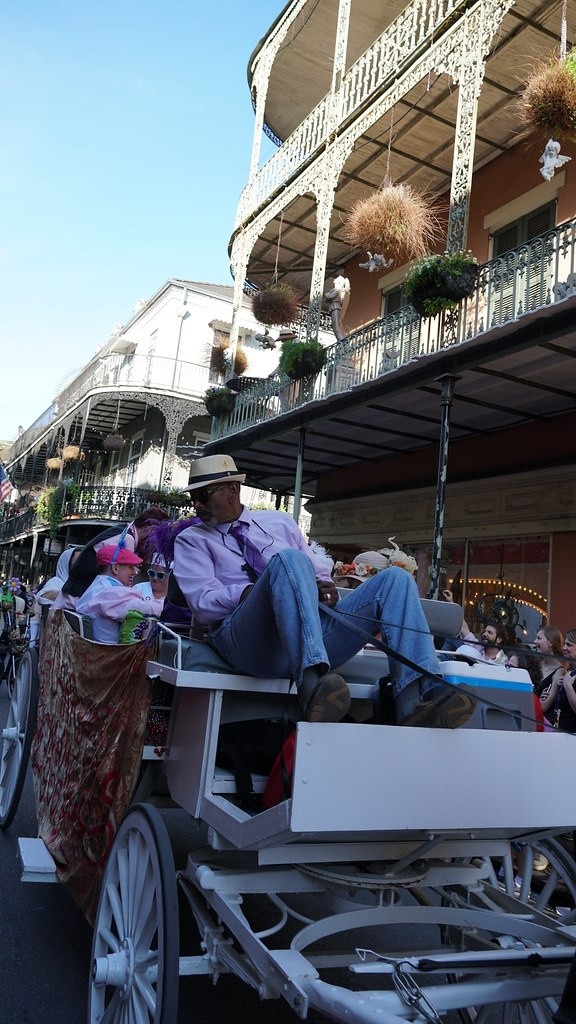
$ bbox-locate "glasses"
[190,483,232,506]
[505,664,521,669]
[147,569,172,580]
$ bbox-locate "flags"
[0,463,19,505]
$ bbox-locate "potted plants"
[397,248,480,317]
[143,484,169,504]
[167,486,189,507]
[278,338,326,379]
[200,386,236,418]
[103,434,125,451]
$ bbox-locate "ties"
[227,521,268,577]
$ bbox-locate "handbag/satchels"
[216,716,297,809]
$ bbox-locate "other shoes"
[533,853,549,871]
[304,672,351,723]
[400,681,478,728]
[498,877,531,899]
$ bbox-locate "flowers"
[7,577,21,591]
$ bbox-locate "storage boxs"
[440,660,535,732]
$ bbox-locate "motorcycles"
[1,607,28,700]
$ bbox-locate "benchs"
[40,604,94,641]
[146,622,389,700]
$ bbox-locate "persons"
[173,455,478,728]
[268,329,301,412]
[443,589,576,925]
[309,536,416,640]
[27,510,172,644]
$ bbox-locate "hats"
[177,454,247,493]
[96,545,144,567]
[332,551,394,583]
[150,553,175,567]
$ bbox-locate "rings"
[327,593,331,599]
